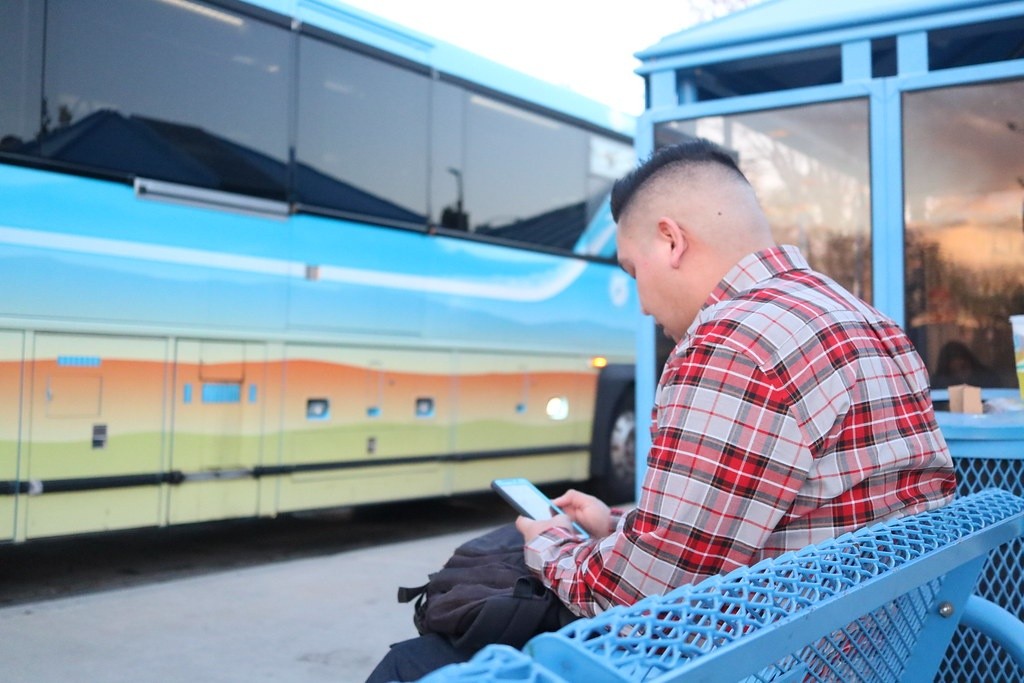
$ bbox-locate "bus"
[0,0,643,545]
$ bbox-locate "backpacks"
[397,520,599,658]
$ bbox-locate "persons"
[928,340,1000,389]
[364,138,957,683]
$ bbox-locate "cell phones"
[491,478,590,543]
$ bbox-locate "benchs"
[387,477,1024,683]
